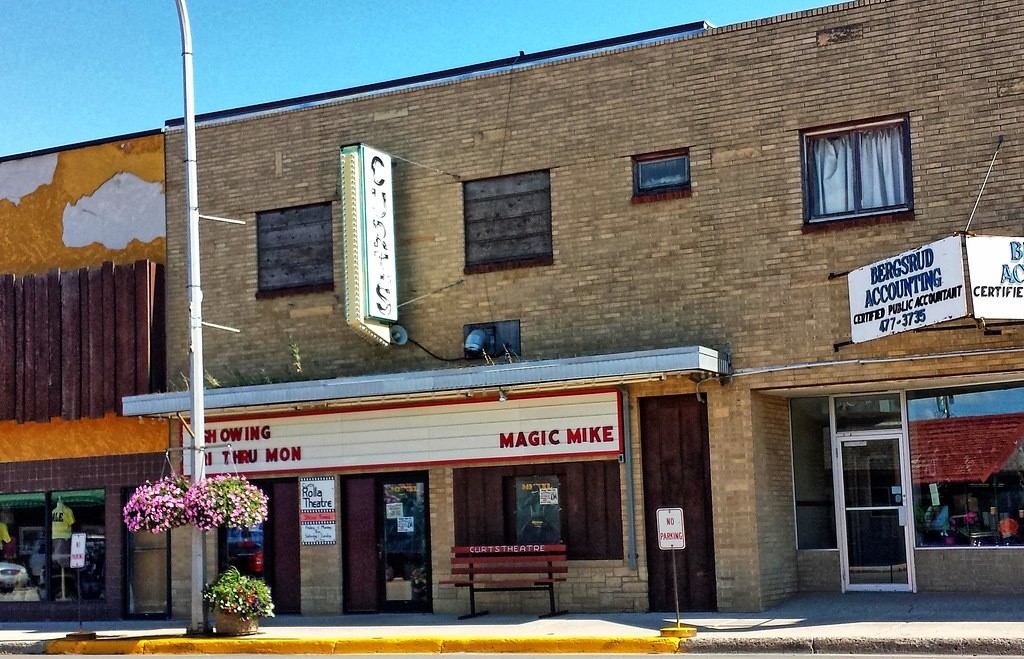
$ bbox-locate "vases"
[213,608,258,636]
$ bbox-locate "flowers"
[121,476,190,534]
[182,475,268,532]
[203,567,275,620]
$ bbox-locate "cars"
[0,562,30,594]
[30,535,106,601]
[386,529,425,580]
[227,530,263,581]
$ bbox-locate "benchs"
[438,544,568,620]
